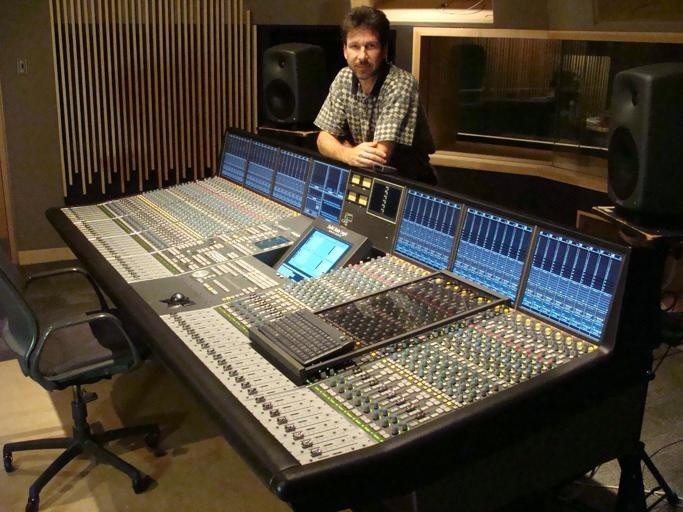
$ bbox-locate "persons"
[310,4,441,187]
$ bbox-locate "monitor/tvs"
[273,215,373,283]
[218,130,350,225]
[391,189,626,343]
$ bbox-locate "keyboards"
[259,308,354,367]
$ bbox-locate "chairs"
[0,248,162,512]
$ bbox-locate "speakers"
[260,42,323,130]
[607,60,683,231]
